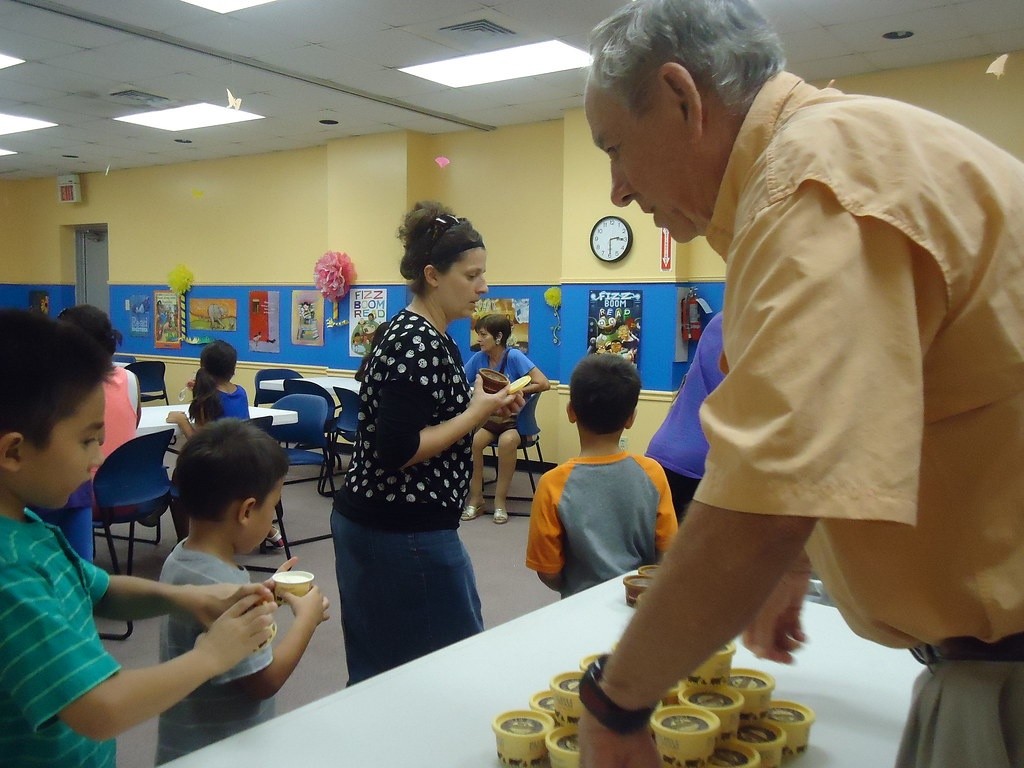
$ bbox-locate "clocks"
[590,215,632,262]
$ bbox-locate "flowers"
[314,250,357,301]
[543,287,562,308]
[166,264,193,294]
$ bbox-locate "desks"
[254,376,367,482]
[137,399,298,549]
[156,567,926,768]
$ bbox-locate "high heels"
[493,509,509,524]
[461,501,486,520]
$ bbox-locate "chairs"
[471,390,548,515]
[247,368,363,495]
[126,360,169,407]
[96,429,180,642]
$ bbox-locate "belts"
[908,634,1024,666]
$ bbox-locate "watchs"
[579,651,659,734]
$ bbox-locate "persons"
[525,354,679,601]
[331,200,526,688]
[0,311,278,768]
[166,340,285,552]
[460,314,550,524]
[579,0,1024,768]
[154,419,332,768]
[57,304,142,521]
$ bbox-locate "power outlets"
[619,436,626,451]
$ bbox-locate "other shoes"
[265,528,284,549]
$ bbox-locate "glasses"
[421,214,460,266]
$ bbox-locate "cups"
[241,597,277,654]
[492,640,815,768]
[272,571,314,605]
[623,565,660,608]
[479,367,509,394]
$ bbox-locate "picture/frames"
[154,290,181,349]
[587,289,643,388]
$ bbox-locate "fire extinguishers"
[681,286,701,341]
